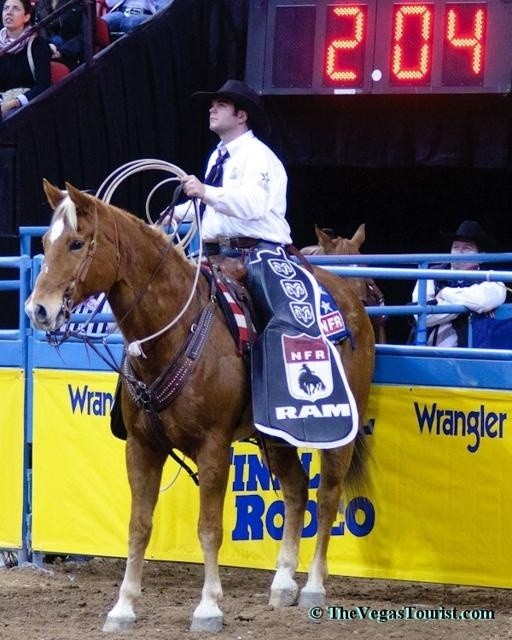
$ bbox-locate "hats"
[193,80,271,138]
[448,221,485,242]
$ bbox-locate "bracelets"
[15,99,21,109]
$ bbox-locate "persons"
[99,0,172,46]
[408,217,509,350]
[150,79,364,448]
[30,0,95,65]
[1,0,54,117]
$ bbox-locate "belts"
[206,238,260,255]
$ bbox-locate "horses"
[23,177,377,636]
[299,223,386,345]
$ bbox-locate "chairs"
[31,0,109,85]
